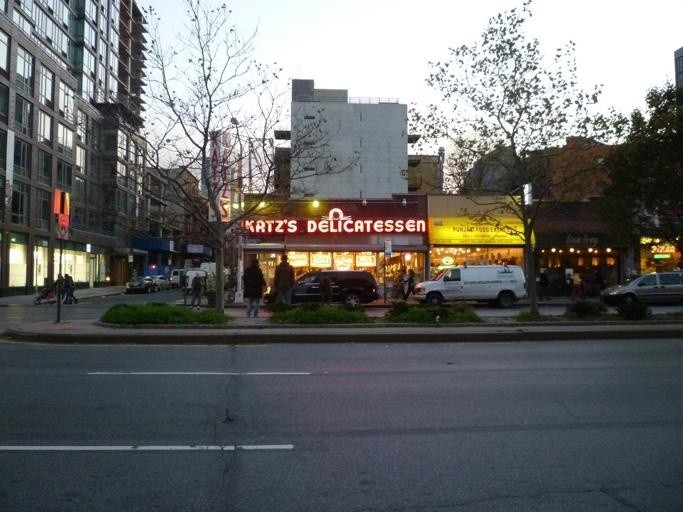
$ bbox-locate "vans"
[411,264,529,307]
[263,270,379,311]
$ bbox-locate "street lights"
[230,118,242,211]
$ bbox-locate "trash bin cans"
[207,290,216,304]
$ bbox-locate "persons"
[189,272,203,311]
[271,254,296,313]
[33,272,79,305]
[535,263,606,301]
[392,265,416,302]
[241,258,268,318]
[318,272,334,306]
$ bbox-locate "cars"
[598,271,683,311]
[125,261,228,296]
